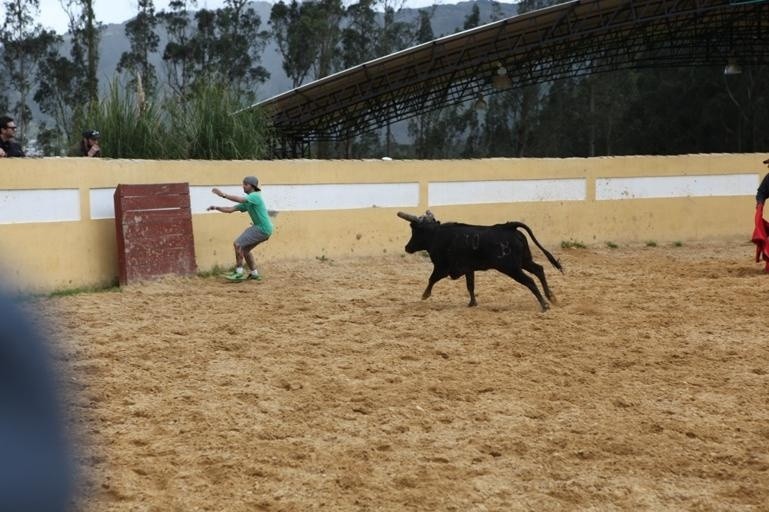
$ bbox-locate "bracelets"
[214,205,217,211]
[222,194,228,198]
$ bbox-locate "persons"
[1,116,43,158]
[205,176,274,282]
[66,129,101,156]
[753,159,769,209]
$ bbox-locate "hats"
[244,176,260,191]
[82,129,100,139]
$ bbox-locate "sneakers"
[225,270,244,281]
[247,274,262,281]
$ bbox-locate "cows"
[397,209,563,312]
